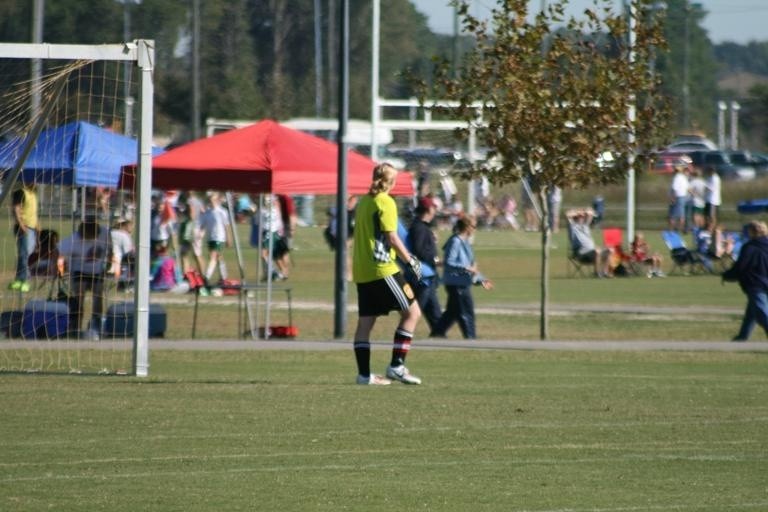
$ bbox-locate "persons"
[12,186,110,295]
[353,162,427,388]
[516,163,563,233]
[720,218,768,343]
[56,223,122,339]
[567,207,666,279]
[109,188,295,298]
[693,216,733,261]
[667,164,723,233]
[320,172,518,284]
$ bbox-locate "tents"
[0,120,163,184]
[115,117,416,199]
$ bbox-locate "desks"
[191,280,293,347]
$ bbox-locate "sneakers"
[8,280,21,290]
[386,365,421,385]
[20,281,30,293]
[357,374,391,386]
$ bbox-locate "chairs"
[563,212,745,286]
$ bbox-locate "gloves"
[407,256,422,281]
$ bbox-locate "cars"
[647,137,768,185]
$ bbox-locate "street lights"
[718,100,729,150]
[732,100,742,150]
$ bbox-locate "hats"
[414,197,437,213]
